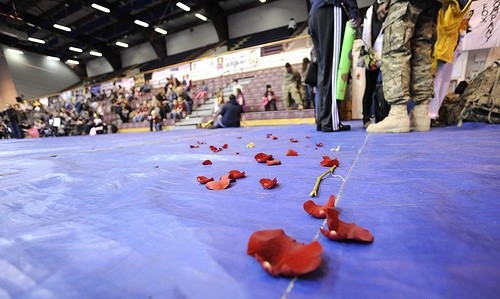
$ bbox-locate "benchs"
[118,62,316,128]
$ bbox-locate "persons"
[430,0,473,130]
[288,17,297,36]
[280,63,304,109]
[307,1,361,132]
[302,58,315,110]
[366,1,442,132]
[359,0,384,130]
[0,60,247,143]
[261,84,282,112]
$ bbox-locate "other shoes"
[338,124,351,130]
[366,104,410,133]
[409,98,431,130]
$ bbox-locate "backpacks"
[439,92,462,127]
[456,59,500,127]
